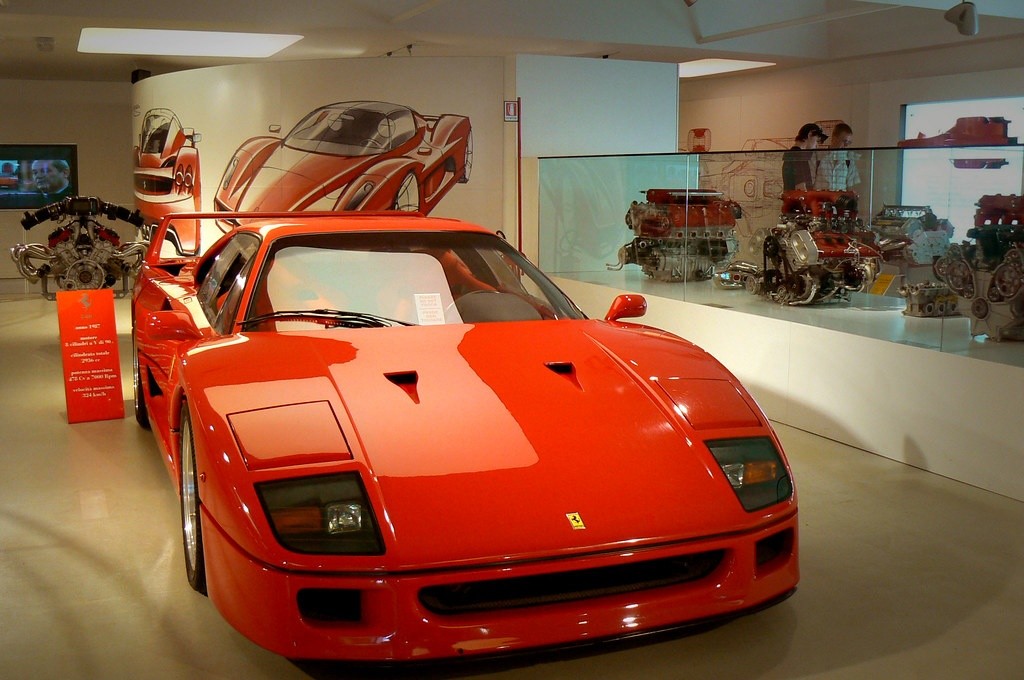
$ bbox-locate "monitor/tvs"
[0,143,77,212]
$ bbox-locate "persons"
[782,122,829,192]
[22,160,71,195]
[814,123,862,192]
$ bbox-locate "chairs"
[393,249,495,324]
[218,257,336,329]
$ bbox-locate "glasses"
[837,136,853,145]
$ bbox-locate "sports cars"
[130,210,801,662]
[1,173,18,188]
